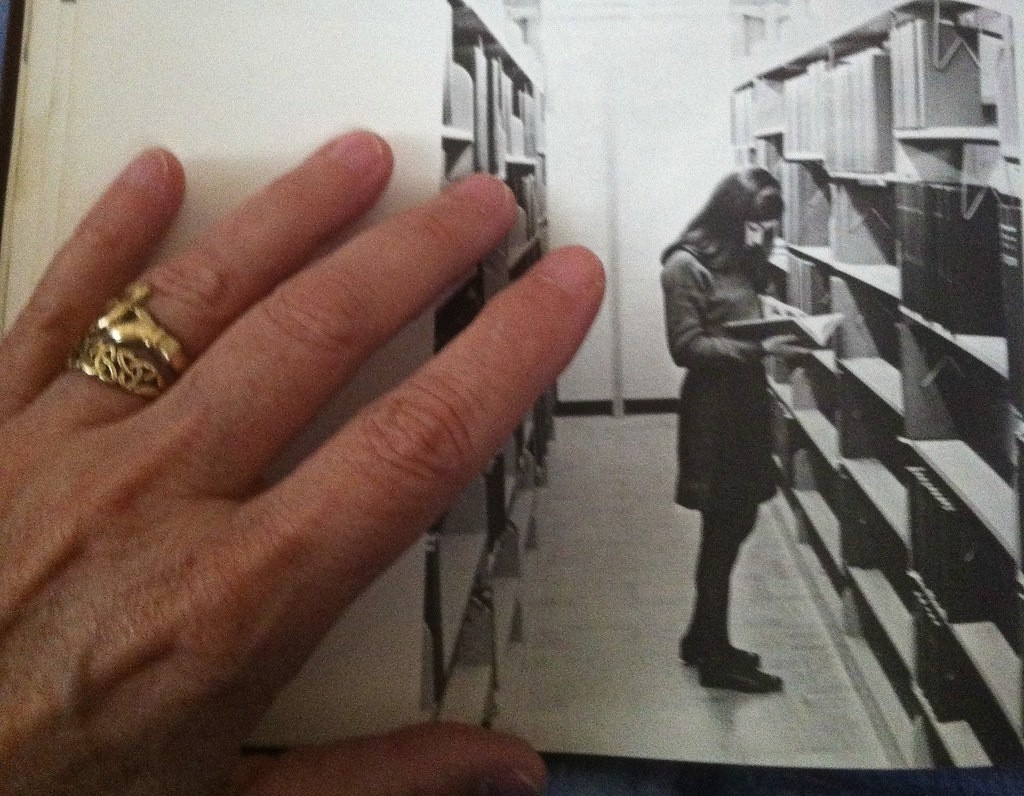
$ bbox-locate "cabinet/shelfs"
[726,0,1024,769]
[0,0,553,757]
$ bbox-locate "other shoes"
[679,637,759,668]
[699,664,783,692]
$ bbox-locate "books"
[722,313,844,349]
[730,18,1023,722]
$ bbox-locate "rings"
[96,282,190,371]
[67,334,167,398]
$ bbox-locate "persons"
[659,169,808,691]
[0,133,606,796]
